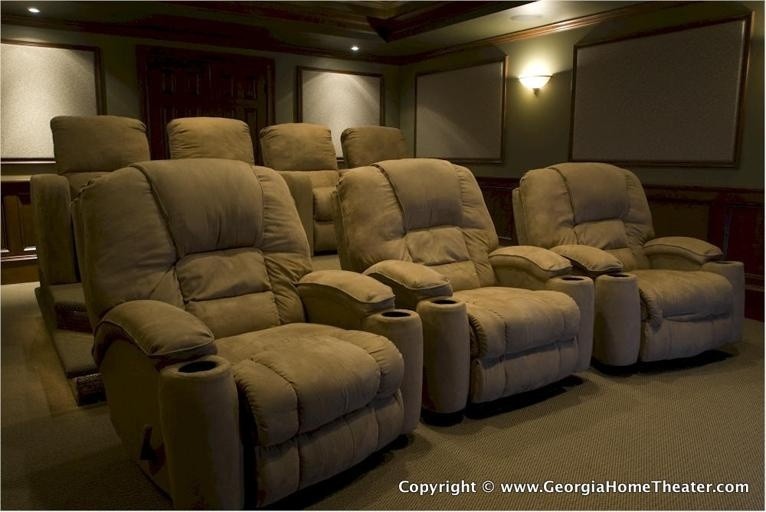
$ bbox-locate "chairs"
[510,163,748,368]
[257,123,342,270]
[164,118,256,164]
[341,126,411,166]
[68,159,424,509]
[32,115,150,405]
[329,158,594,425]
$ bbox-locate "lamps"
[518,75,551,96]
